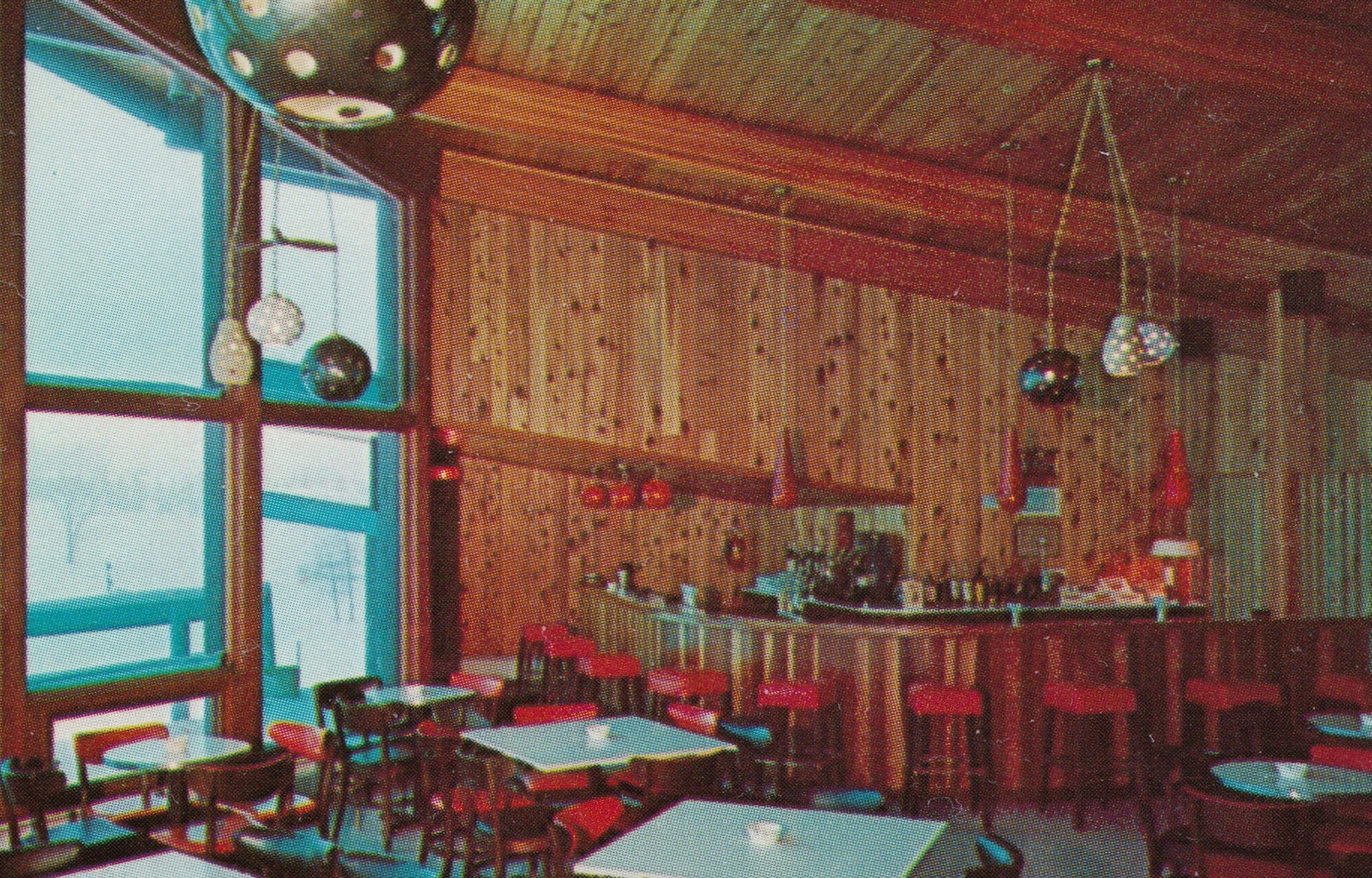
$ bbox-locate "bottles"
[787,541,1064,612]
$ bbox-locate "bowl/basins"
[1279,763,1306,778]
[166,737,188,751]
[586,723,611,740]
[406,685,424,696]
[748,821,782,847]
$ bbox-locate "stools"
[516,620,1371,833]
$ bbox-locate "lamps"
[207,111,373,399]
[999,142,1030,509]
[766,186,805,513]
[1019,60,1176,405]
[1165,179,1195,508]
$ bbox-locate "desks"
[1309,712,1372,748]
[55,849,254,878]
[1215,759,1371,800]
[464,717,740,800]
[103,735,254,828]
[572,799,957,878]
[366,686,475,737]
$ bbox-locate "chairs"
[1135,755,1371,878]
[963,831,1024,878]
[723,721,776,807]
[3,676,720,878]
